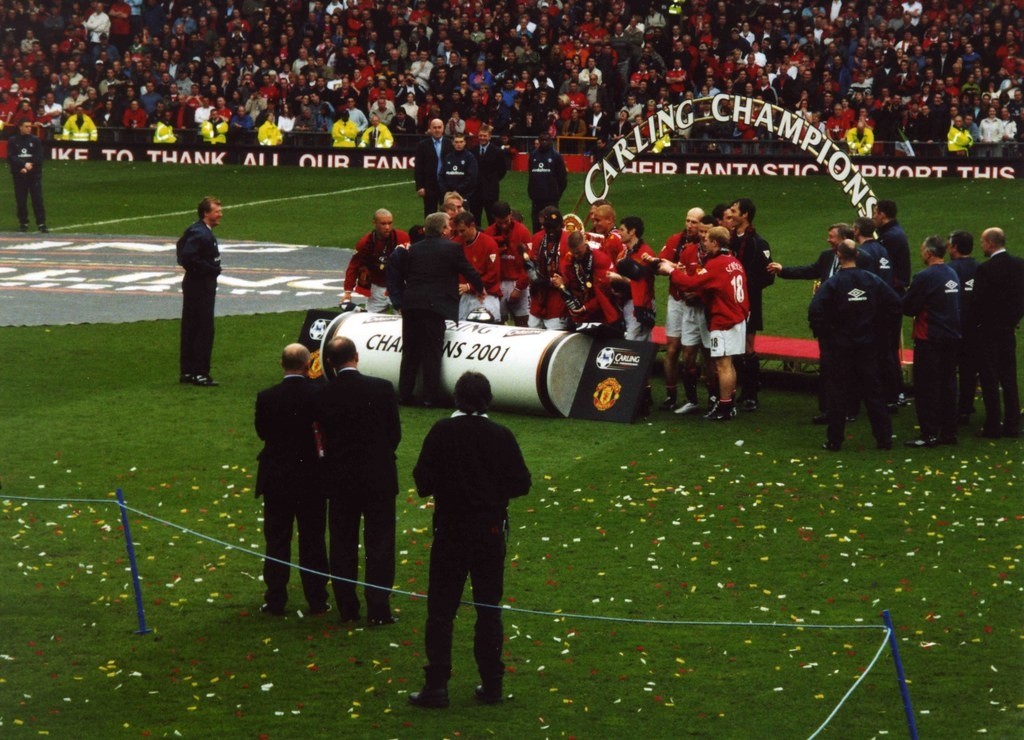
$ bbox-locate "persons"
[338,190,775,421]
[0,1,1023,157]
[470,126,507,230]
[314,337,402,627]
[407,372,533,709]
[414,118,454,221]
[766,198,1024,454]
[6,118,50,233]
[175,198,223,387]
[439,132,478,214]
[528,132,568,236]
[254,343,331,615]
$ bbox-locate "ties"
[480,148,485,158]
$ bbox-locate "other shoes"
[310,602,333,613]
[476,686,483,697]
[368,616,400,626]
[702,401,737,421]
[39,224,49,234]
[178,372,219,387]
[877,441,894,449]
[707,392,718,410]
[735,396,757,412]
[259,602,270,615]
[644,386,653,407]
[19,222,28,232]
[340,612,361,622]
[822,440,840,452]
[937,434,957,444]
[999,427,1020,438]
[662,390,677,411]
[905,434,938,447]
[887,403,898,415]
[971,427,1000,438]
[673,402,702,415]
[813,410,858,423]
[407,691,423,709]
